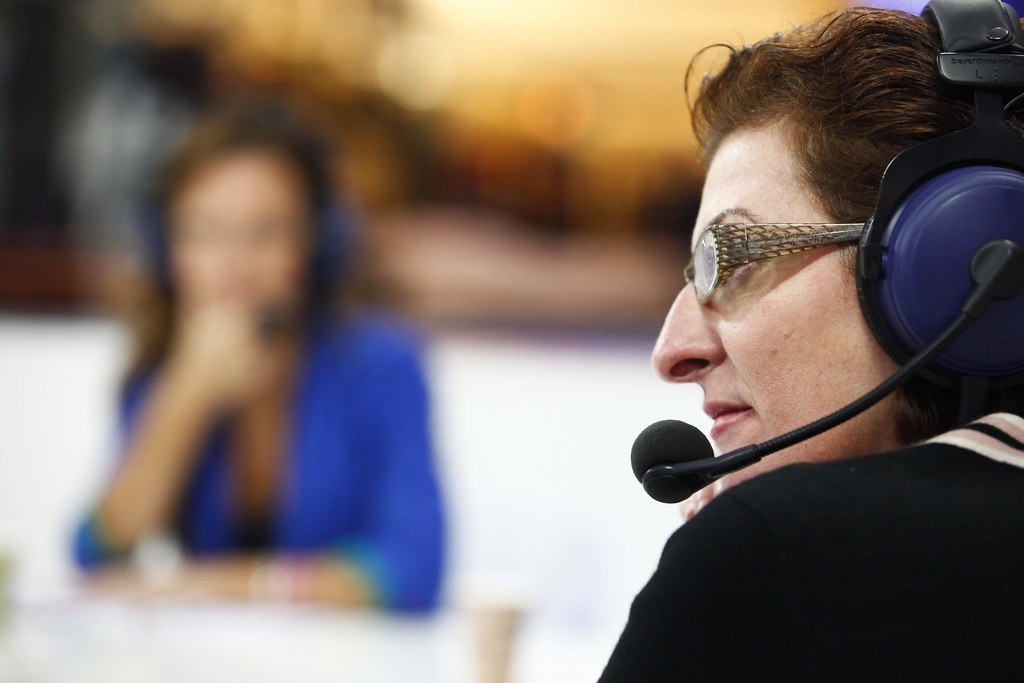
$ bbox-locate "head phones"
[857,0,1024,383]
[138,128,353,300]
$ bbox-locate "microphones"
[257,303,311,335]
[630,240,1024,503]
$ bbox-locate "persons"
[69,112,444,616]
[596,1,1024,683]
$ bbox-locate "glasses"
[684,223,866,304]
